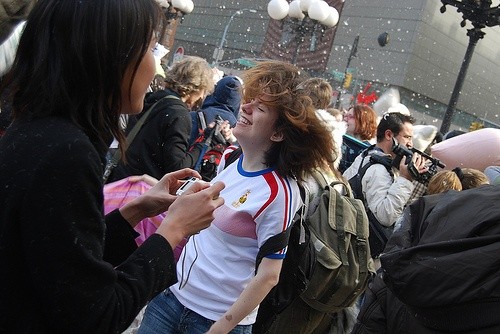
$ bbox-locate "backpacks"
[290,168,373,313]
[350,144,392,207]
[198,111,233,177]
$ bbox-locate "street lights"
[267,0,340,68]
[156,0,194,45]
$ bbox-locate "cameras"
[176,176,220,200]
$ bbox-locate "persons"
[0,0,226,334]
[0,0,500,334]
[138,60,341,333]
